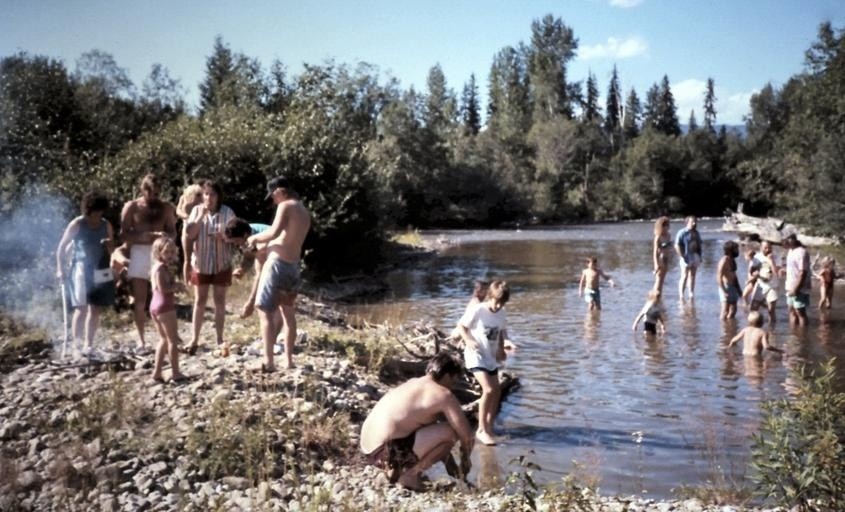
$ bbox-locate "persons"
[584,312,603,343]
[449,281,490,348]
[57,191,113,364]
[783,339,813,393]
[223,176,311,375]
[111,174,238,384]
[740,357,769,392]
[578,256,616,312]
[631,215,844,356]
[459,278,511,446]
[358,351,477,493]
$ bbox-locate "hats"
[263,176,287,200]
